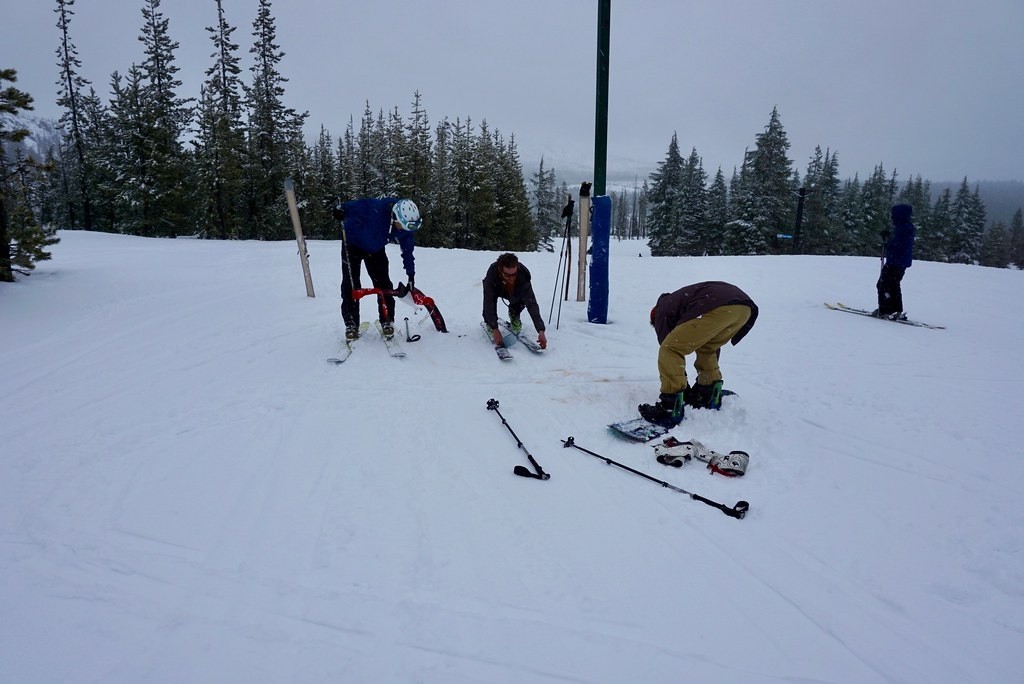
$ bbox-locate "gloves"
[332,204,346,220]
[407,274,414,292]
[879,230,891,240]
[878,242,887,251]
[681,377,699,404]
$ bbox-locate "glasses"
[406,218,422,230]
[501,271,518,278]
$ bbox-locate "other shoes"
[873,308,887,317]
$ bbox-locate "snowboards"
[608,384,738,440]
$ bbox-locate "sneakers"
[485,322,495,341]
[507,312,522,331]
[346,324,359,341]
[643,392,686,423]
[691,380,724,411]
[381,321,394,338]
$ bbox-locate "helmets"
[392,198,420,232]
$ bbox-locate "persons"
[871,203,916,321]
[638,281,759,428]
[482,253,547,349]
[337,200,422,341]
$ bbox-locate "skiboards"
[323,317,408,364]
[825,299,945,331]
[480,318,548,363]
